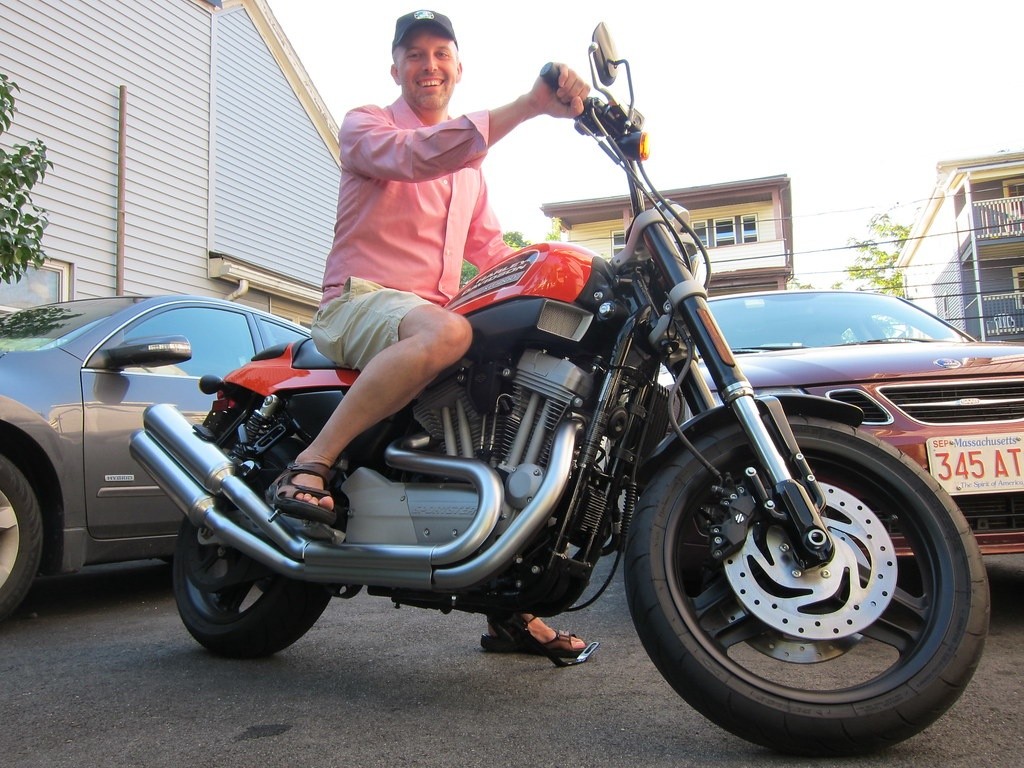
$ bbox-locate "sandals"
[264,462,337,524]
[480,614,591,657]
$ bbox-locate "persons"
[272,9,590,658]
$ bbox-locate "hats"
[392,10,459,54]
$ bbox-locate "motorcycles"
[128,22,992,759]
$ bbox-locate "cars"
[0,295,350,624]
[657,290,1024,573]
